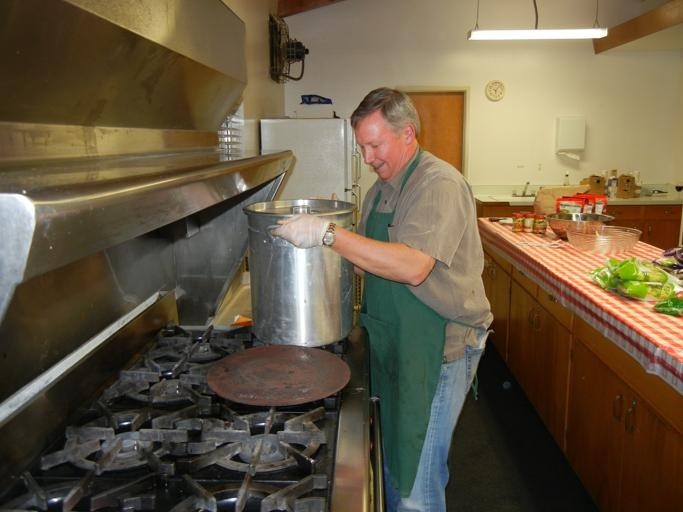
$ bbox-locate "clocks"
[486,80,506,102]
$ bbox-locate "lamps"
[465,0,608,42]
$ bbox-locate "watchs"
[321,221,336,249]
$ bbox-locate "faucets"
[523,182,530,195]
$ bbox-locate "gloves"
[270,214,332,250]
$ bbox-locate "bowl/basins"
[566,225,642,254]
[546,212,616,240]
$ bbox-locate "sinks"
[489,196,535,206]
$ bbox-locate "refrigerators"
[260,117,362,234]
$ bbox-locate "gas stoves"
[0,323,348,511]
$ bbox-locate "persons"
[268,87,496,510]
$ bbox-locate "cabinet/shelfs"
[508,265,576,451]
[565,316,683,512]
[481,240,513,364]
[605,205,683,251]
